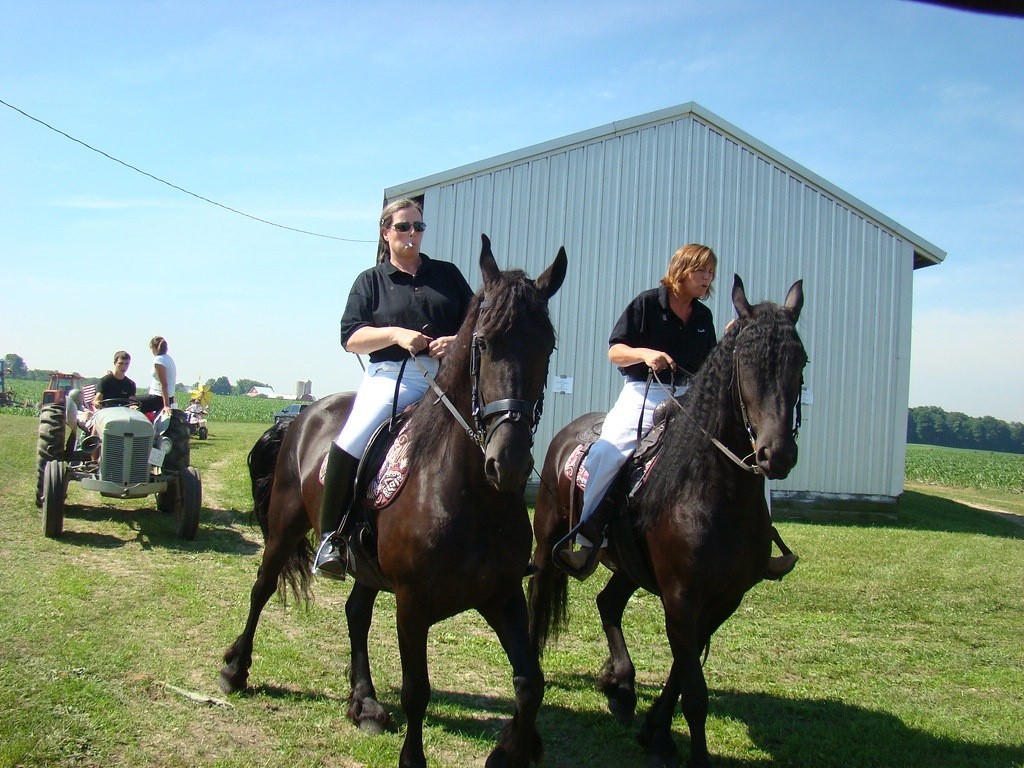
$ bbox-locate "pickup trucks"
[274,403,308,424]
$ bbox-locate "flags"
[82,382,98,402]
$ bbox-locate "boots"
[314,440,360,579]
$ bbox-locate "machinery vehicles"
[35,396,202,541]
[43,373,83,408]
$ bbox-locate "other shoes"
[561,545,603,582]
[769,553,799,580]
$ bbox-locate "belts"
[625,374,689,386]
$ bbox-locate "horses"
[221,234,568,768]
[524,274,811,768]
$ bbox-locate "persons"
[313,198,540,576]
[562,244,798,578]
[187,399,208,419]
[137,336,177,416]
[90,351,136,461]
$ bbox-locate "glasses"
[387,221,427,231]
[195,402,200,404]
